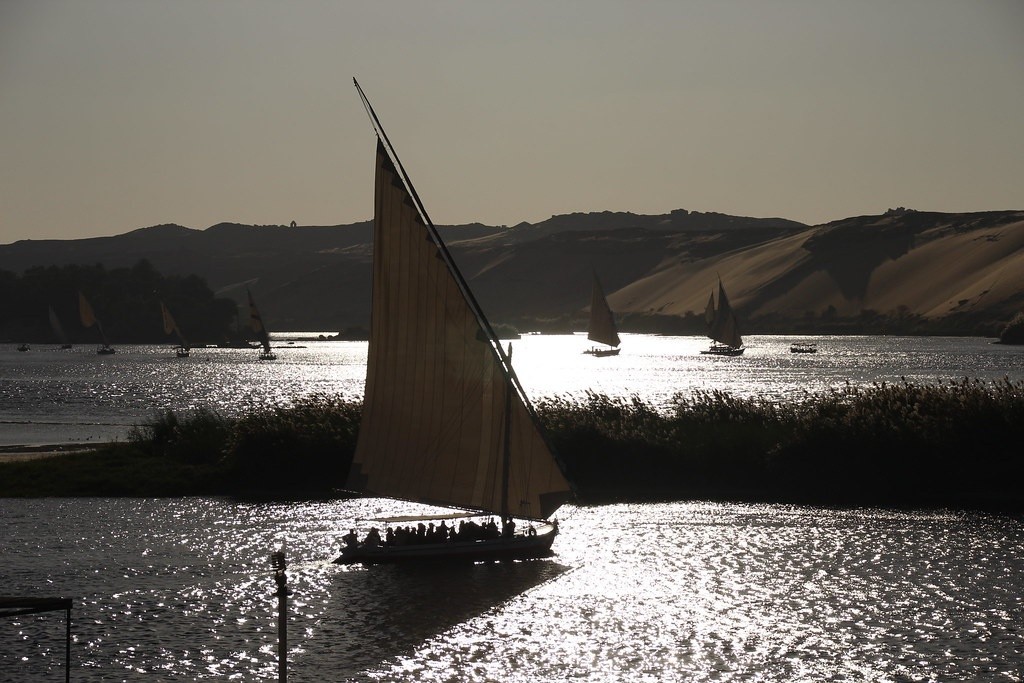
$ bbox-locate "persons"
[343,517,516,547]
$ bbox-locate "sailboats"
[700,273,746,356]
[583,270,622,358]
[337,75,577,561]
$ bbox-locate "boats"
[96,344,116,354]
[790,343,818,353]
[259,350,277,360]
[176,348,190,358]
